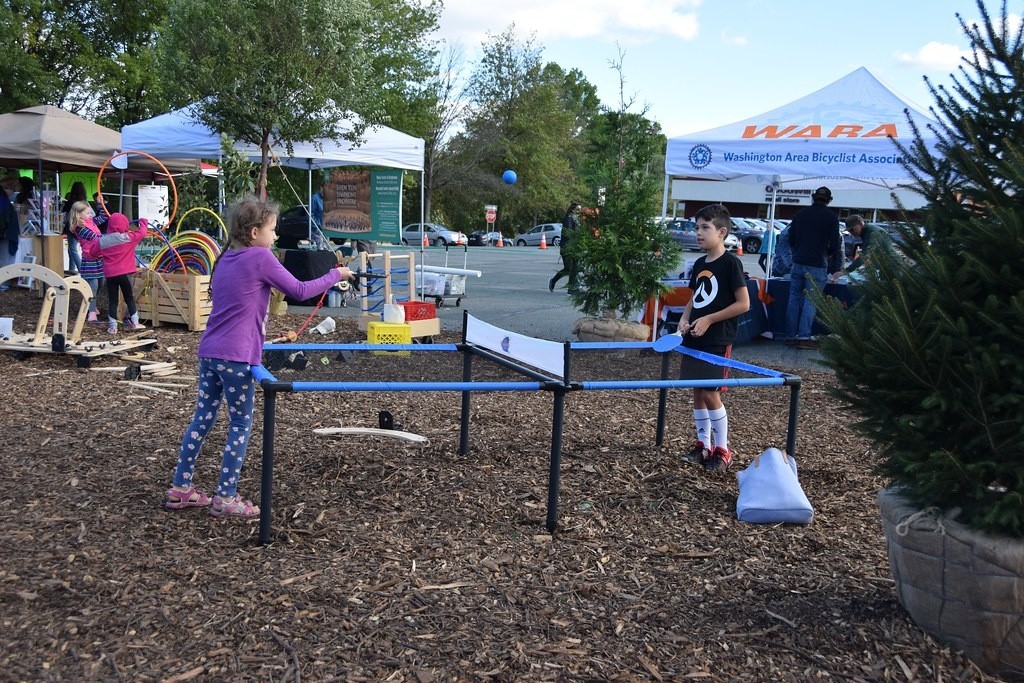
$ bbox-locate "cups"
[784,274,791,279]
[837,275,852,285]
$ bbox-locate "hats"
[814,186,833,201]
[845,214,862,231]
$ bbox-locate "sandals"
[210,492,261,517]
[166,487,212,509]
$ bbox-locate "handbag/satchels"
[736,448,813,524]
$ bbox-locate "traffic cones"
[655,250,660,256]
[537,231,549,249]
[424,233,430,246]
[735,239,745,256]
[495,231,504,248]
[457,230,463,247]
[854,246,860,262]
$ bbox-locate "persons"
[548,202,582,296]
[833,214,901,282]
[677,204,751,472]
[0,174,35,267]
[772,187,845,348]
[165,197,356,517]
[93,192,111,234]
[350,239,374,295]
[90,212,148,334]
[62,181,87,272]
[69,195,108,321]
[757,223,776,274]
[306,182,329,248]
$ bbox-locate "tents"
[0,104,201,297]
[652,66,974,343]
[120,70,425,302]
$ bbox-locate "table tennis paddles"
[652,323,690,352]
[329,266,387,279]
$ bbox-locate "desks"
[9,235,65,290]
[765,276,865,340]
[249,310,803,535]
[281,250,337,306]
[636,275,768,346]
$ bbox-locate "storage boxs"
[367,321,412,358]
[445,275,466,295]
[397,301,436,321]
[415,272,445,296]
[117,270,214,331]
[405,317,441,338]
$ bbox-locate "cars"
[395,223,469,245]
[274,204,347,247]
[512,222,564,246]
[644,215,931,253]
[466,229,515,246]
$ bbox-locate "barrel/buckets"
[0,318,14,338]
[328,289,342,307]
[684,259,697,279]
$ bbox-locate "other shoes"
[87,312,98,322]
[64,270,77,276]
[785,338,798,345]
[0,285,9,291]
[797,339,821,349]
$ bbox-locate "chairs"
[335,246,353,267]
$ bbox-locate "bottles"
[827,273,840,280]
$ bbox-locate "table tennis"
[502,169,517,184]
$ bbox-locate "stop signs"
[486,210,496,223]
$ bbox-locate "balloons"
[503,170,517,185]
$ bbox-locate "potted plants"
[555,110,684,356]
[800,0,1024,683]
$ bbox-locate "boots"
[107,316,118,335]
[129,311,146,329]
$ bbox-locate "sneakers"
[681,441,714,465]
[700,446,733,473]
